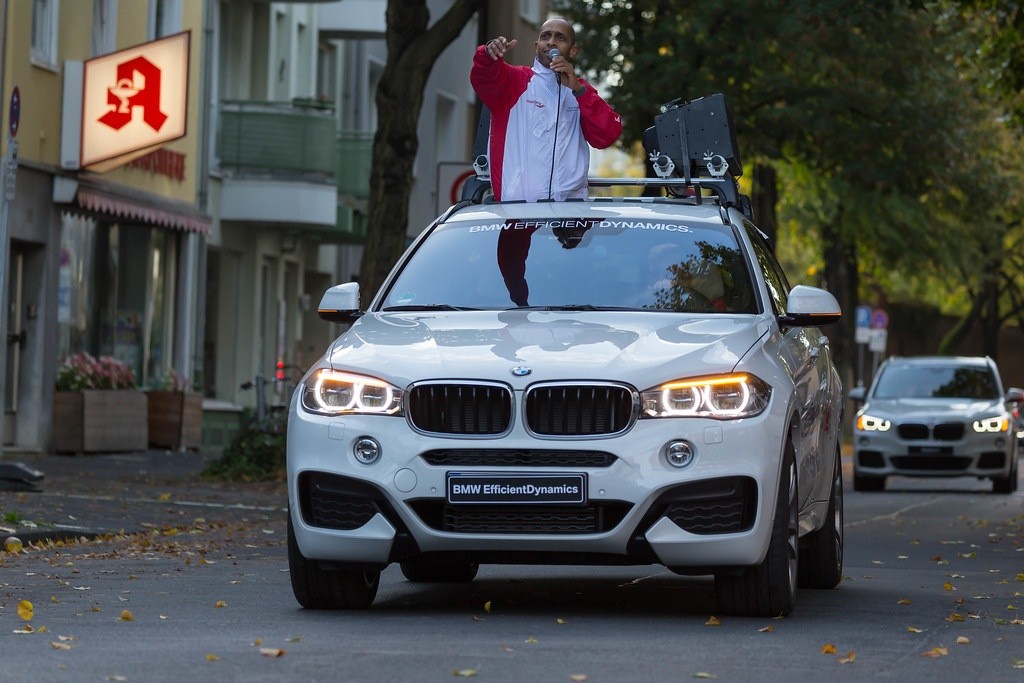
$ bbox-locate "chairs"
[691,260,726,301]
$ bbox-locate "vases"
[53,392,148,455]
[142,391,202,451]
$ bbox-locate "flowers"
[147,371,189,390]
[54,352,136,392]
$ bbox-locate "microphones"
[549,49,561,86]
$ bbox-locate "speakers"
[643,92,744,177]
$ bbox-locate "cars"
[287,177,843,618]
[849,355,1024,495]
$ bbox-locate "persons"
[470,18,624,201]
[627,243,716,313]
